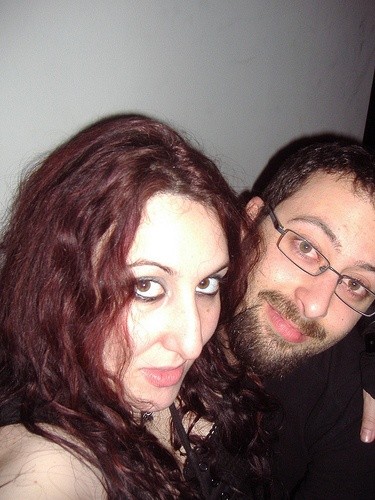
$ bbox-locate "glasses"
[264,199,375,317]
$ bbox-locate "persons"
[214,132,375,500]
[0,112,375,500]
